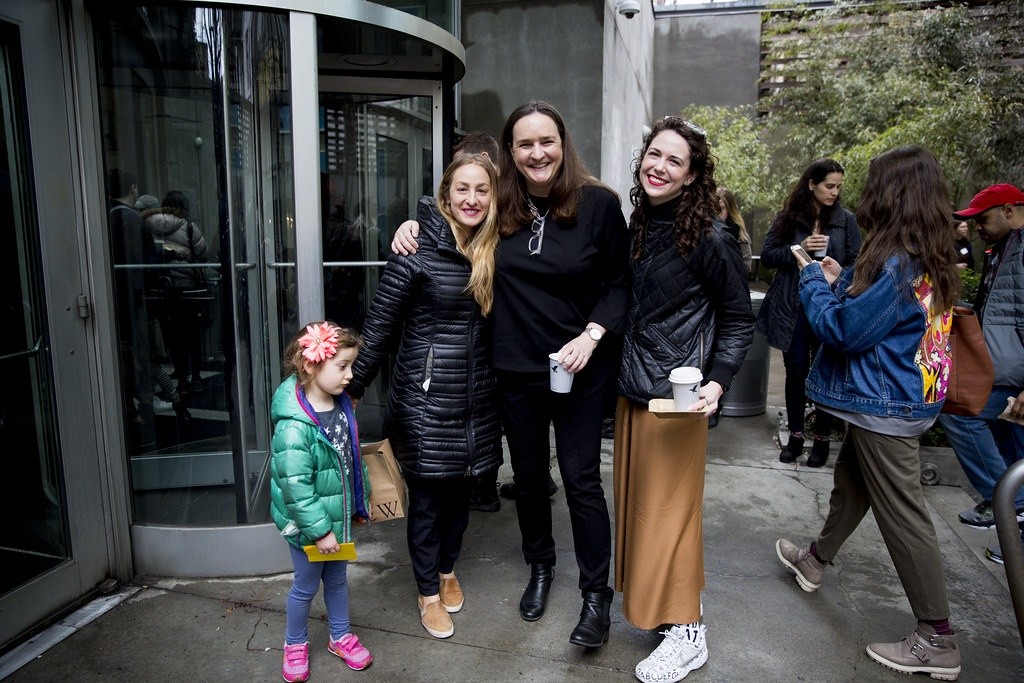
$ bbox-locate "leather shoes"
[520,562,557,620]
[570,587,615,647]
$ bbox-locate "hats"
[952,183,1024,221]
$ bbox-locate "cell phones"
[791,244,812,265]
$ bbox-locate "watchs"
[583,328,602,342]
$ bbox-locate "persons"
[776,145,961,683]
[757,160,861,467]
[324,198,356,263]
[268,320,371,681]
[347,102,632,649]
[950,220,976,274]
[708,187,751,429]
[938,183,1024,566]
[609,117,756,682]
[103,164,206,449]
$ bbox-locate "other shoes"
[806,437,831,467]
[779,435,805,462]
[435,572,464,613]
[462,480,502,512]
[417,594,454,638]
[499,466,557,497]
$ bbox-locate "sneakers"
[777,539,828,592]
[958,498,1024,531]
[282,640,310,683]
[985,532,1024,564]
[327,632,373,670]
[867,620,961,680]
[634,622,709,683]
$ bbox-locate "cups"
[814,236,830,257]
[668,367,703,412]
[549,353,575,393]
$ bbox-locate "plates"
[648,398,709,419]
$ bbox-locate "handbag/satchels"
[354,440,409,523]
[939,306,994,416]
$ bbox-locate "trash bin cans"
[722,290,770,417]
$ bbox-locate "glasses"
[528,216,545,256]
[664,115,707,145]
